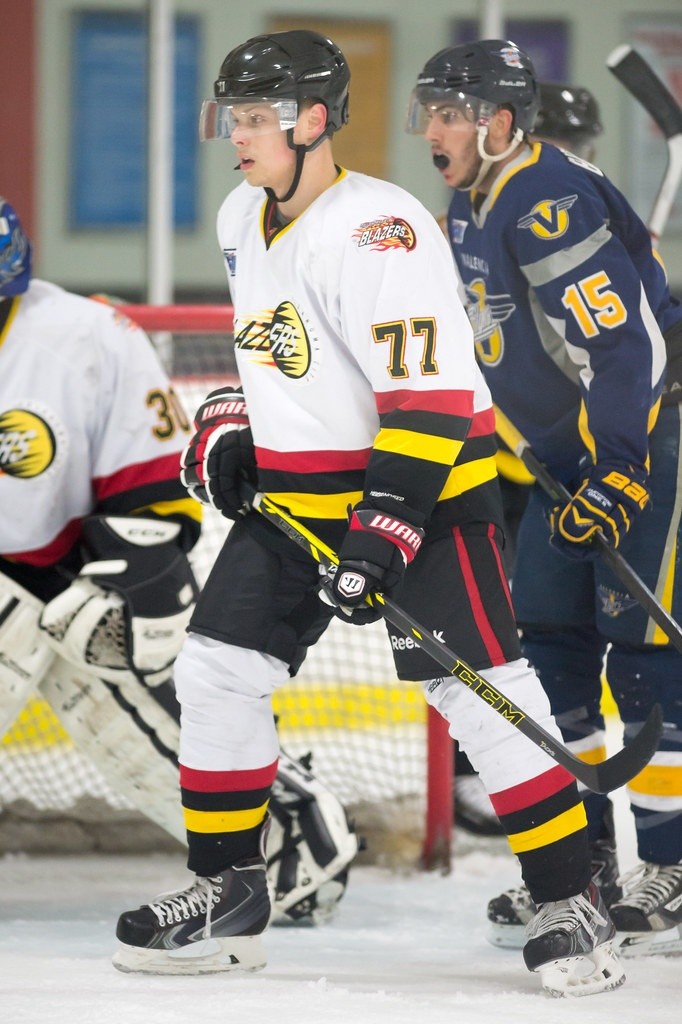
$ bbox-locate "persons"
[1,198,357,926]
[413,40,682,954]
[111,29,624,998]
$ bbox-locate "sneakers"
[113,812,271,977]
[486,840,622,948]
[608,859,682,958]
[520,861,625,997]
[452,740,510,849]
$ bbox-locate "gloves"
[316,497,426,624]
[180,386,257,521]
[543,466,653,563]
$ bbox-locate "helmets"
[412,38,541,128]
[540,81,603,143]
[0,199,31,297]
[214,27,352,130]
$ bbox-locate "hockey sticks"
[492,401,682,649]
[604,40,682,256]
[235,474,665,797]
